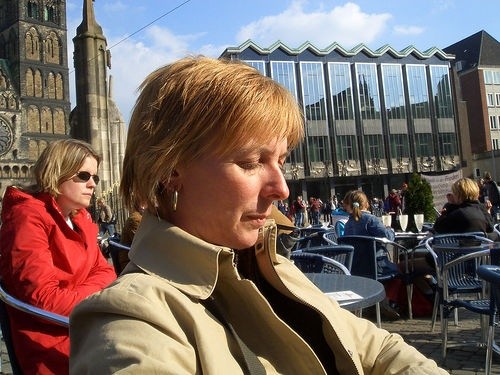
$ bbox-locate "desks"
[395,231,429,264]
[302,273,385,328]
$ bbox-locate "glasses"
[77,171,99,185]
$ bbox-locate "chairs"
[425,234,494,332]
[323,232,340,246]
[291,245,354,273]
[290,253,363,318]
[107,232,131,279]
[441,249,490,359]
[336,236,413,328]
[0,288,71,375]
[478,264,500,375]
[429,232,489,327]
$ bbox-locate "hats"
[390,189,398,193]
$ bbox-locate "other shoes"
[381,305,400,321]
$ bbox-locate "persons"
[278,172,500,230]
[0,139,117,375]
[97,198,114,259]
[421,177,495,317]
[344,191,415,318]
[69,55,449,375]
[119,201,145,271]
